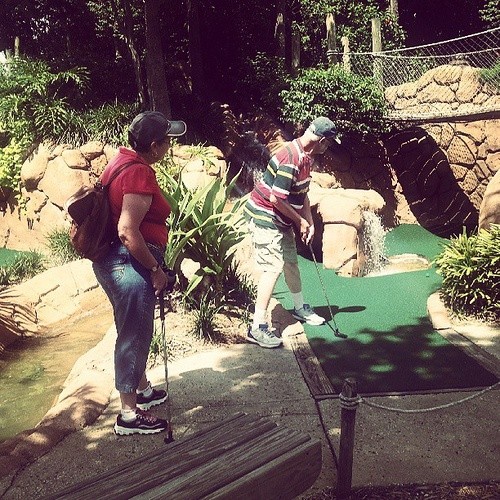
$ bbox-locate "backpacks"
[63,162,155,263]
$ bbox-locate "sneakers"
[112,407,168,434]
[137,388,168,410]
[247,322,283,349]
[293,303,326,326]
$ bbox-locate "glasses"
[326,138,333,144]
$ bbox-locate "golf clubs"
[159,291,175,444]
[309,241,348,338]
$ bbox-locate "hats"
[128,109,187,141]
[308,116,342,145]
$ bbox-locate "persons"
[242,116,342,348]
[89,111,188,435]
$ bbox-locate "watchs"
[148,265,160,272]
